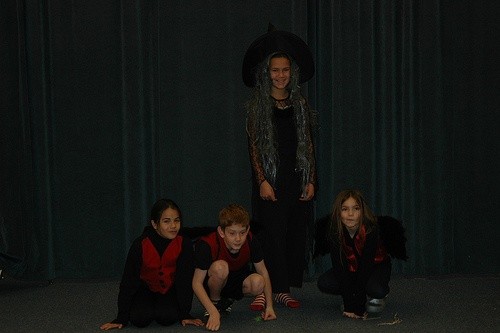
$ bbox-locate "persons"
[192,205,277,331]
[100,199,205,330]
[315,190,390,320]
[245,53,315,311]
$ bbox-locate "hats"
[242,21,315,87]
